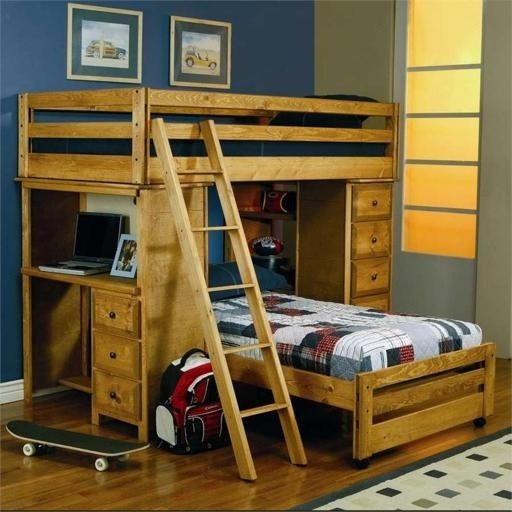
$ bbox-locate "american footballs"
[249,235,284,256]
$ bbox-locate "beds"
[208,255,497,471]
[16,85,497,446]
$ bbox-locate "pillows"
[266,88,382,129]
[208,261,295,302]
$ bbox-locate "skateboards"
[6,420,150,471]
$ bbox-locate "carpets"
[282,425,512,511]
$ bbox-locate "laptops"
[38,211,123,275]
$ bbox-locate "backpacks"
[153,347,231,456]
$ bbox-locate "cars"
[87,40,125,60]
[183,45,216,71]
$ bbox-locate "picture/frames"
[108,233,138,280]
[168,14,233,90]
[65,2,144,84]
